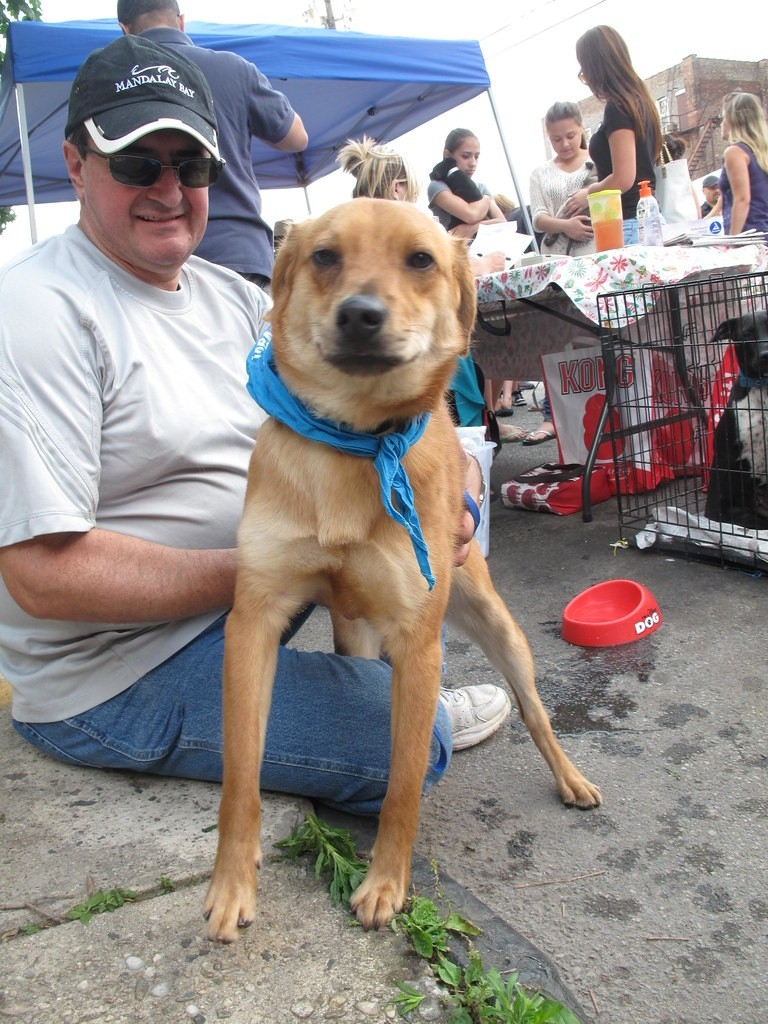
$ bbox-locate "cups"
[586,189,625,253]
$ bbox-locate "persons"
[560,25,663,247]
[334,127,546,442]
[117,0,310,292]
[0,35,511,815]
[654,92,768,248]
[495,380,552,417]
[520,102,598,445]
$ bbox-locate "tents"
[0,18,491,245]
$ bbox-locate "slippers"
[485,424,528,442]
[528,399,546,411]
[493,401,514,417]
[524,430,557,446]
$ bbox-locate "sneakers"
[440,684,511,751]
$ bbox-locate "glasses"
[578,67,587,85]
[81,147,226,190]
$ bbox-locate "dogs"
[193,131,614,945]
[696,312,768,538]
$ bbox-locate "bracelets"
[462,447,487,507]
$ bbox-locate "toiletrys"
[636,180,664,247]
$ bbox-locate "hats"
[703,175,720,187]
[64,33,225,165]
[118,0,180,35]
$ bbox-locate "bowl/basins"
[562,578,663,648]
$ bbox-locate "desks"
[471,244,759,430]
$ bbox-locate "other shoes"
[511,389,527,406]
[518,381,534,390]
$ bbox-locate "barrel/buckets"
[465,441,496,556]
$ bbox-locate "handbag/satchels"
[653,142,703,225]
[540,336,712,494]
[502,461,613,516]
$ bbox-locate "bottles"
[636,180,663,248]
